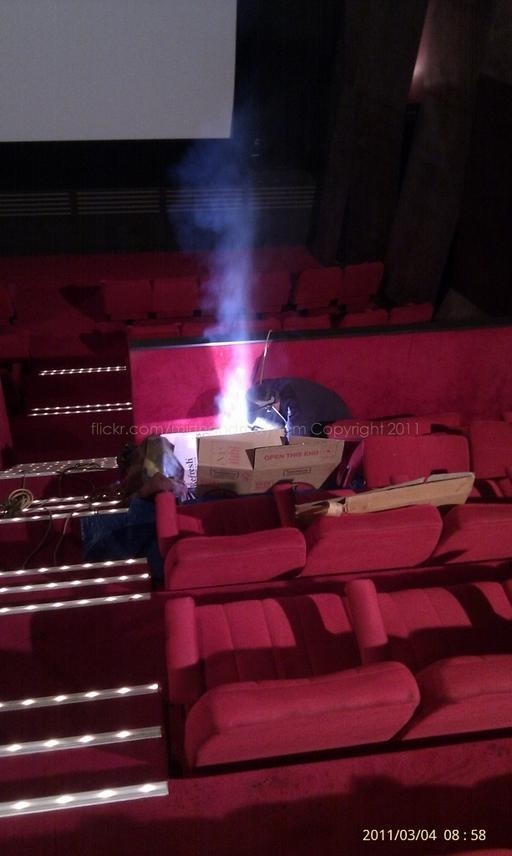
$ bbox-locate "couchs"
[97,259,512,776]
